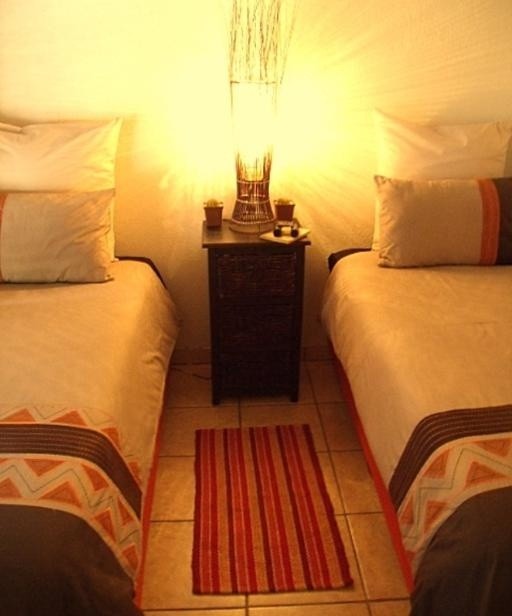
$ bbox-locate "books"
[258,224,311,245]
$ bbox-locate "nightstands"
[202,217,311,405]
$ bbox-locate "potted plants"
[273,197,296,221]
[203,198,224,228]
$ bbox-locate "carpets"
[191,424,354,595]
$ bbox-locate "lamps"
[228,0,293,236]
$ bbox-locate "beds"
[0,256,179,616]
[316,247,512,615]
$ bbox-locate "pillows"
[372,107,512,268]
[0,117,125,285]
